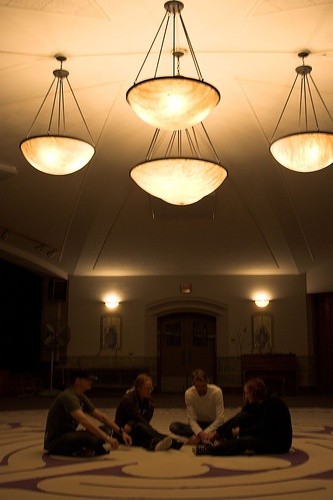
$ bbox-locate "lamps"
[179,275,193,295]
[125,0,221,130]
[268,51,332,173]
[129,122,228,206]
[19,55,97,176]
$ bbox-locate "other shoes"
[151,437,173,452]
[177,436,191,446]
[103,443,114,452]
[192,444,206,456]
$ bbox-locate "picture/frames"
[101,315,121,350]
[252,313,274,348]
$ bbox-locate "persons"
[168,367,224,445]
[112,373,189,451]
[191,378,292,456]
[44,369,132,457]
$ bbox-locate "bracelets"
[117,427,124,436]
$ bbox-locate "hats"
[70,366,99,380]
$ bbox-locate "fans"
[38,317,71,396]
